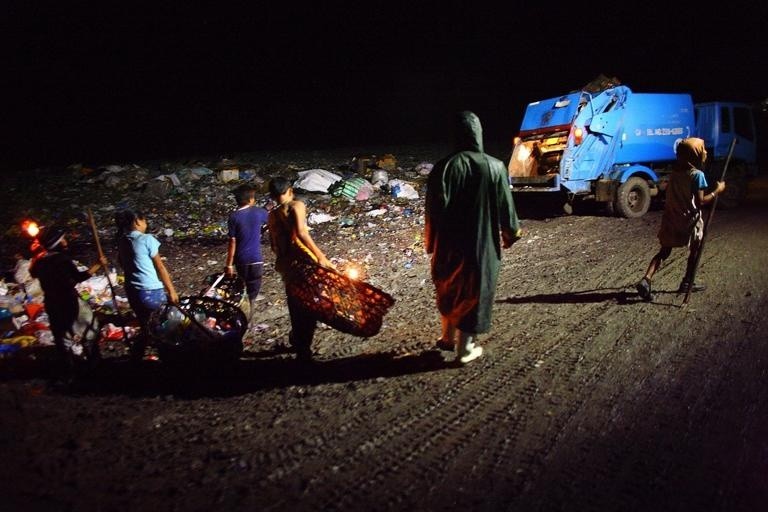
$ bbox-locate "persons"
[268,177,335,359]
[637,138,726,300]
[116,209,179,328]
[224,184,268,303]
[29,229,108,345]
[425,111,522,365]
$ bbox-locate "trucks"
[507,85,759,219]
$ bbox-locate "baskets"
[198,271,251,320]
[284,258,395,339]
[90,309,146,368]
[153,295,248,368]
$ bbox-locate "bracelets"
[226,266,232,268]
[711,192,716,200]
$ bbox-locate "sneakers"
[679,279,706,293]
[636,277,654,301]
[435,338,484,364]
